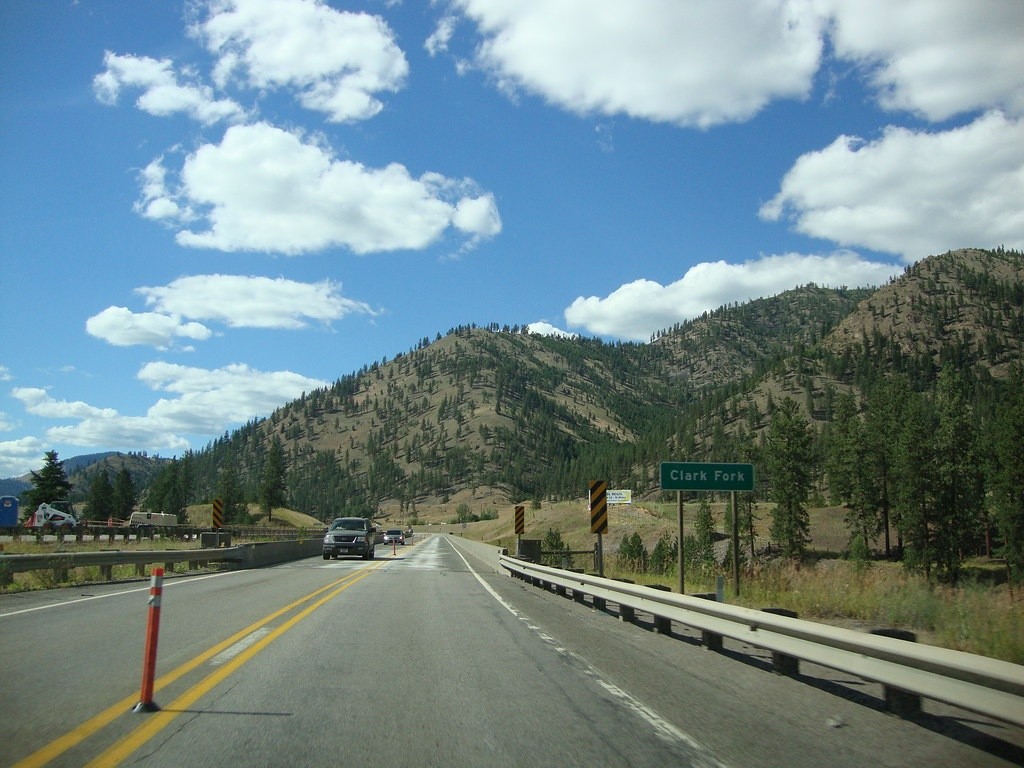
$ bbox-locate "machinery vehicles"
[0,495,178,530]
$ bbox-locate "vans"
[322,515,376,560]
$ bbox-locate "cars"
[383,528,404,545]
